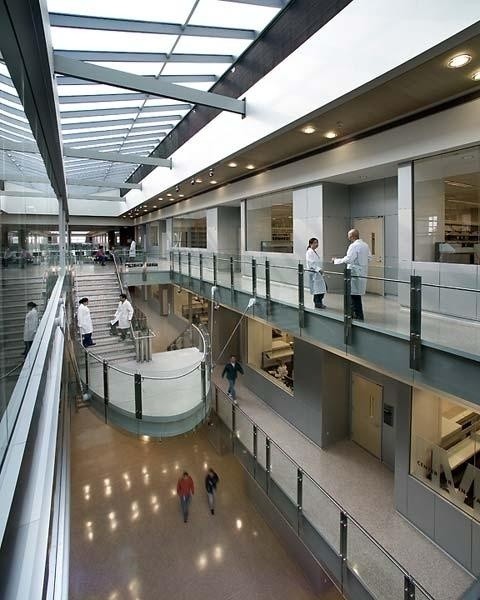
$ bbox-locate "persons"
[306,238,328,308]
[21,302,38,360]
[128,238,136,267]
[96,246,108,266]
[77,298,96,347]
[222,355,244,406]
[205,468,220,515]
[177,472,194,523]
[110,293,134,342]
[2,247,13,268]
[19,247,33,269]
[332,229,373,322]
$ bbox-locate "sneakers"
[184,509,214,523]
[227,392,238,406]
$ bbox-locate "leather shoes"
[83,339,95,348]
[110,332,126,342]
[315,304,363,322]
[22,351,26,355]
